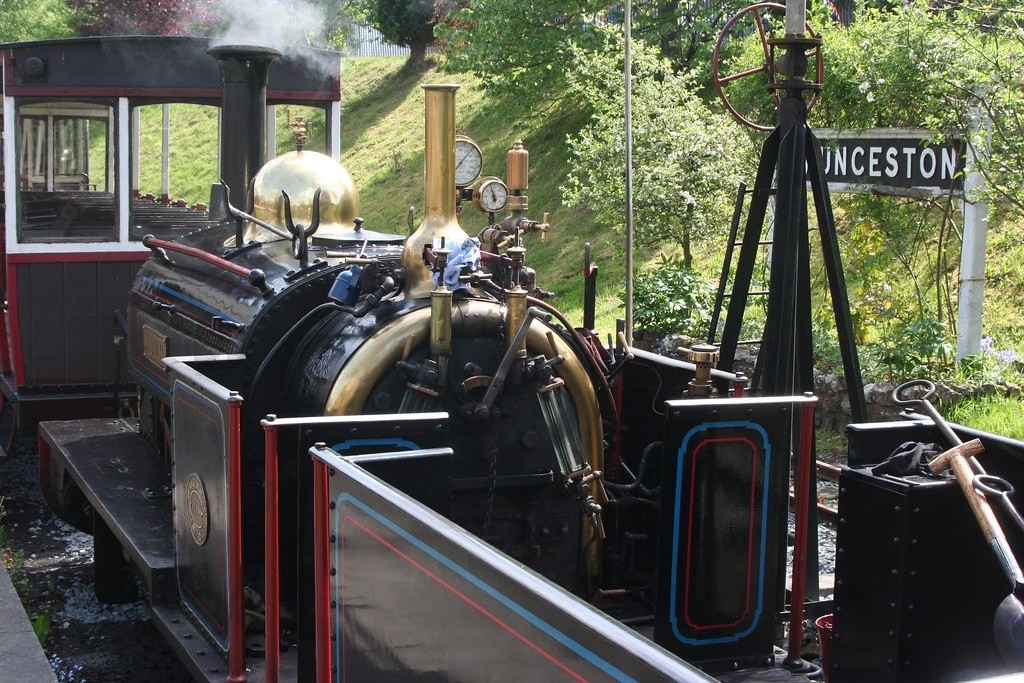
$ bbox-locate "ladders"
[705,180,779,396]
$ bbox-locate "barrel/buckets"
[815,613,834,683]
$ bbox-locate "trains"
[1,2,1022,683]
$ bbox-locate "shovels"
[927,438,1024,675]
